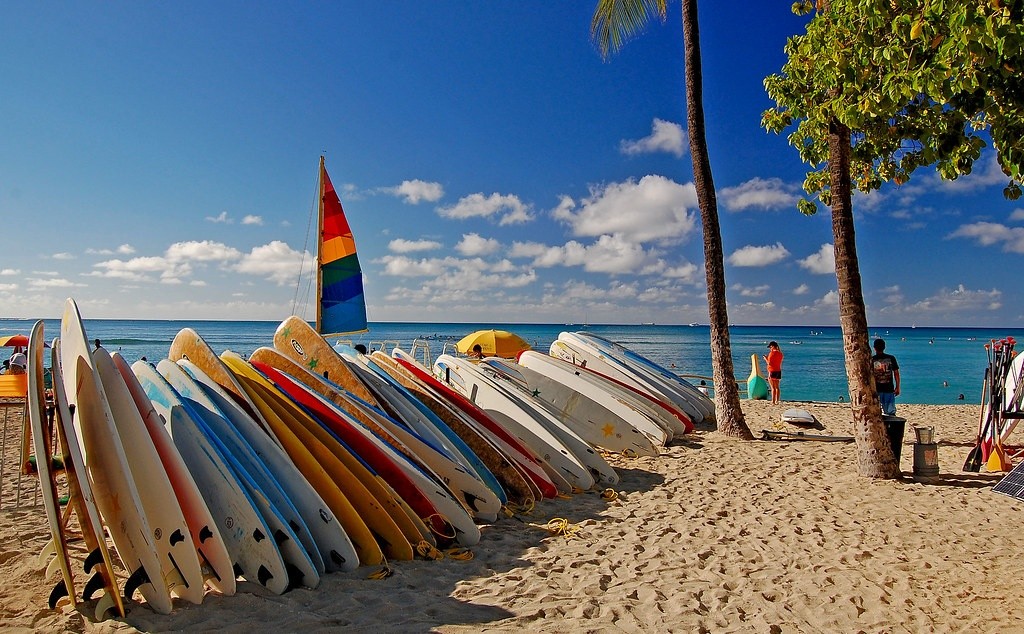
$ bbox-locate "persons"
[811,330,823,335]
[763,341,783,405]
[354,344,366,355]
[367,347,377,355]
[696,380,708,396]
[416,333,464,343]
[942,381,948,386]
[92,339,104,354]
[670,364,676,367]
[958,394,965,400]
[795,340,799,343]
[839,395,843,402]
[873,328,982,346]
[469,344,485,359]
[3,353,26,375]
[871,339,900,416]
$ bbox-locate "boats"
[746,353,769,399]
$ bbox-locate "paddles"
[962,335,1017,474]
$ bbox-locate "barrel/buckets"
[914,426,935,444]
[912,444,939,483]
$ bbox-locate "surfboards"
[58,298,175,613]
[164,327,286,448]
[271,313,388,417]
[782,408,816,424]
[25,293,717,621]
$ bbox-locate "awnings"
[0,334,50,347]
[454,329,532,357]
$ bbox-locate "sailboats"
[277,153,370,356]
